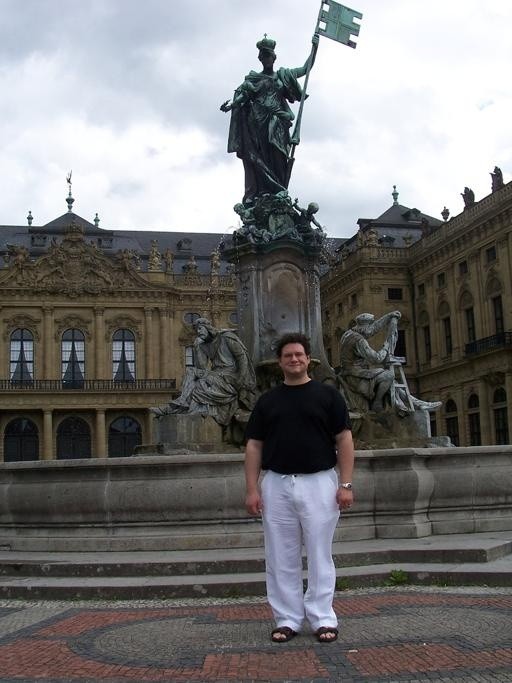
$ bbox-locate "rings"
[348,505,351,507]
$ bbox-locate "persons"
[243,333,355,643]
[219,33,319,204]
[148,317,256,426]
[337,310,443,413]
[232,197,327,240]
[5,238,221,275]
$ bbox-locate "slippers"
[314,626,339,643]
[270,627,299,643]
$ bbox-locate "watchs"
[340,482,352,489]
